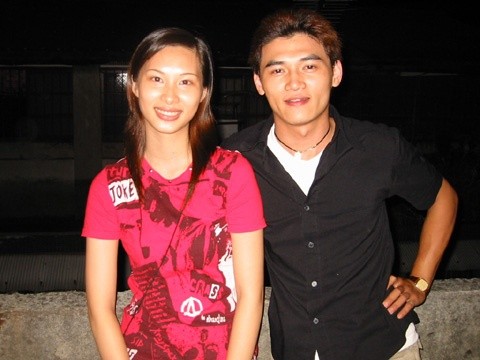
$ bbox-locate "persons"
[217,7,458,360]
[80,27,267,360]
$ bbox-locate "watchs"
[407,276,431,296]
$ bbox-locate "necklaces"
[275,117,331,154]
